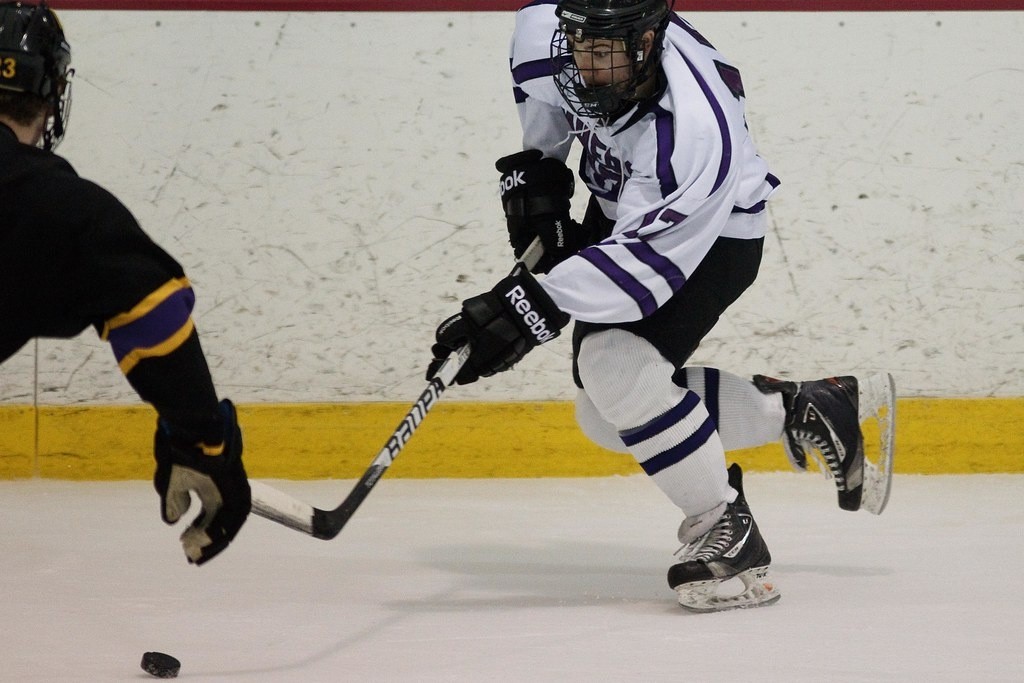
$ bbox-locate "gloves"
[154,397,254,566]
[427,260,572,386]
[495,147,575,273]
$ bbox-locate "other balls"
[138,649,183,680]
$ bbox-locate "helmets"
[0,3,74,154]
[550,0,671,120]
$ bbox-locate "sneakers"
[754,371,894,514]
[668,462,782,609]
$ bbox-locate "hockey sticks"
[244,235,548,547]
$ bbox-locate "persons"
[0,0,252,566]
[496,0,896,609]
